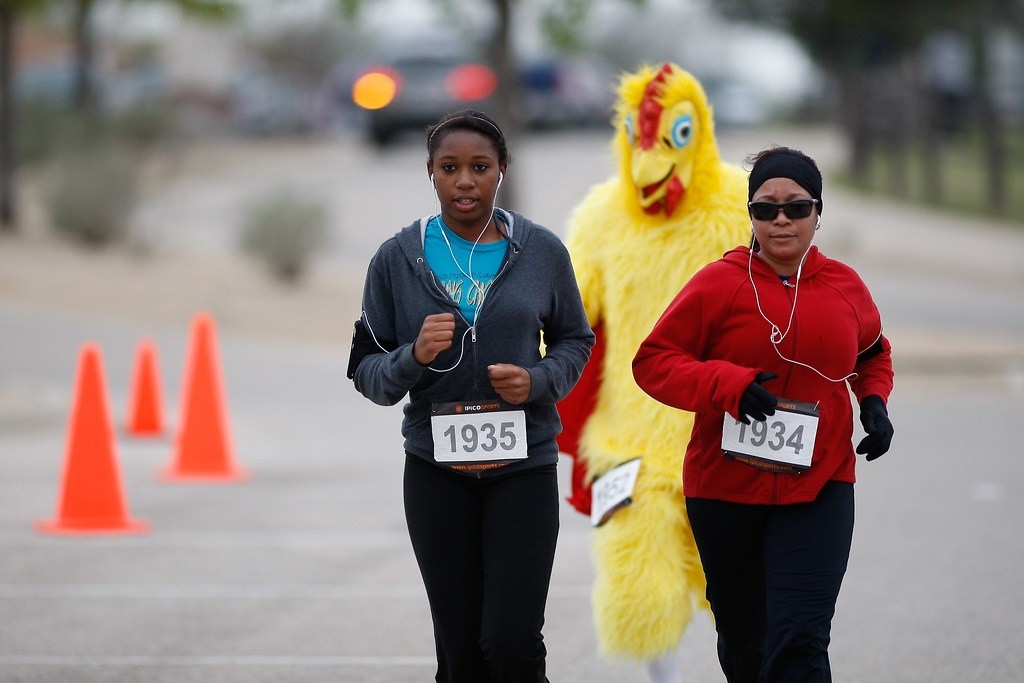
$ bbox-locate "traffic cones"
[32,345,156,537]
[126,349,172,439]
[149,308,254,490]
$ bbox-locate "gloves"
[855,395,894,461]
[738,370,778,426]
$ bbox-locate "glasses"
[747,199,819,220]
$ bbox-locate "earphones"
[750,221,754,232]
[816,215,820,230]
[497,172,503,187]
[430,174,436,189]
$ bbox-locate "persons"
[346,110,600,682]
[631,146,897,682]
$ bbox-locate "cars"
[361,42,499,147]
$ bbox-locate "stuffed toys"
[539,56,757,682]
